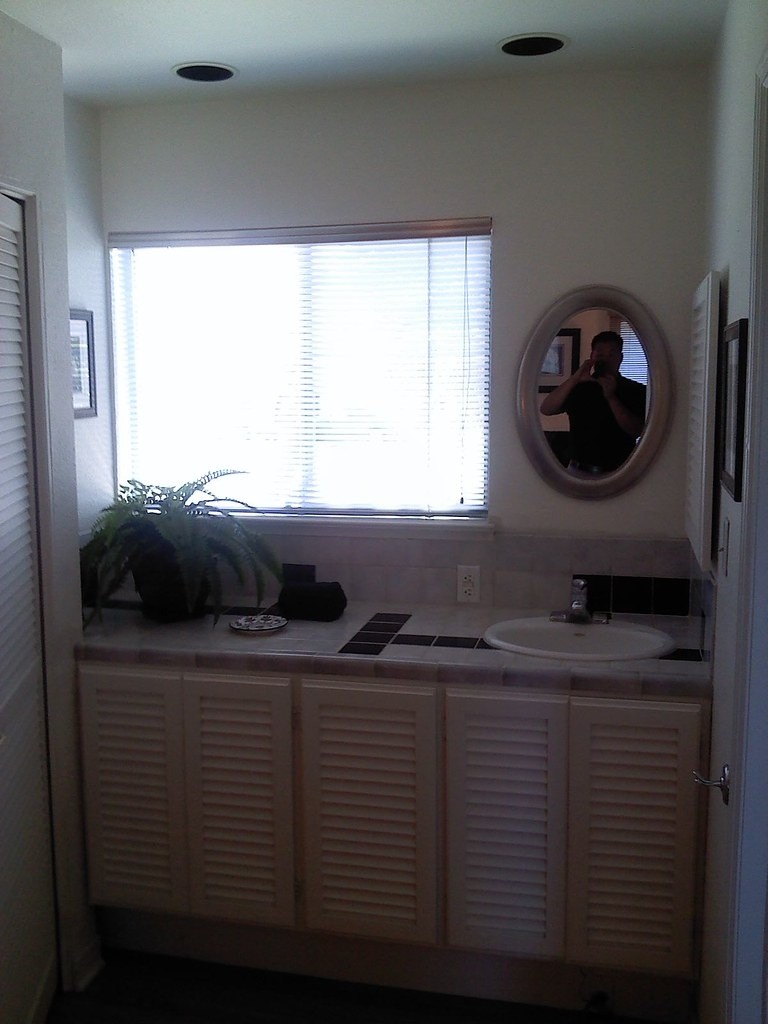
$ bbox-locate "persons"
[540,331,648,478]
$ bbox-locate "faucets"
[548,578,609,625]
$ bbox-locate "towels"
[279,580,348,622]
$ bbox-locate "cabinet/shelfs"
[75,659,712,1012]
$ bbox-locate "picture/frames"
[716,315,751,502]
[538,326,582,395]
[69,307,97,419]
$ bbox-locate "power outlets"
[456,565,481,604]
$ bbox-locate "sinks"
[483,616,677,662]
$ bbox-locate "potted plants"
[79,469,286,633]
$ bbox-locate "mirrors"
[514,283,678,504]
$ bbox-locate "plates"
[228,615,288,635]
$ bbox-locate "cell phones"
[593,359,607,379]
